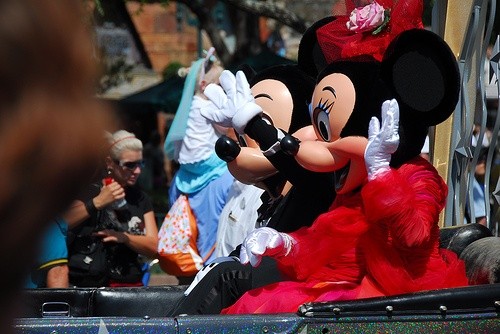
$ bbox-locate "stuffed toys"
[220,0,470,312]
[169,66,337,317]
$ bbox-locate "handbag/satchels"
[67,225,111,286]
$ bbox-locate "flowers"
[346,0,392,36]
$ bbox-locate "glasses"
[113,159,146,168]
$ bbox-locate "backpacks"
[156,194,216,277]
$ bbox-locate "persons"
[168,56,238,285]
[464,150,487,226]
[22,221,69,288]
[64,130,159,286]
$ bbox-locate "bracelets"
[85,200,98,216]
